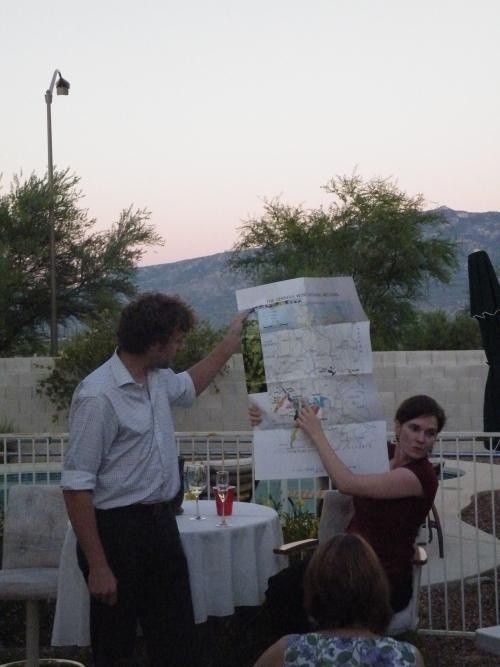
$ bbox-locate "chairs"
[0,482,85,666]
[273,490,427,637]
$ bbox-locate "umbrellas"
[466,250,500,452]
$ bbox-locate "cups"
[213,486,236,517]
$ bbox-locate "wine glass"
[215,471,233,528]
[186,464,209,521]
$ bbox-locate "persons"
[58,292,250,667]
[254,534,425,667]
[254,394,445,650]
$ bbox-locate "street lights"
[45,67,71,354]
